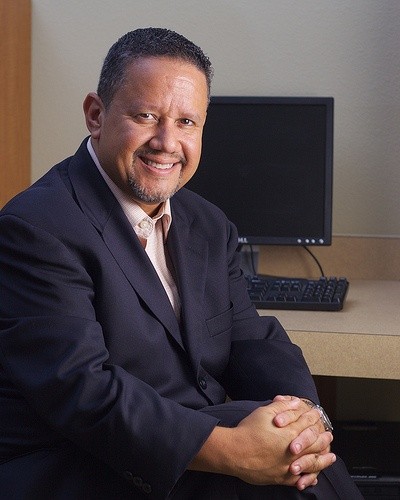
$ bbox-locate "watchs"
[302,399,333,431]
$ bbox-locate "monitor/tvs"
[182,96,334,245]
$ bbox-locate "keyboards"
[247,276,349,311]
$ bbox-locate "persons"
[0,27,361,500]
